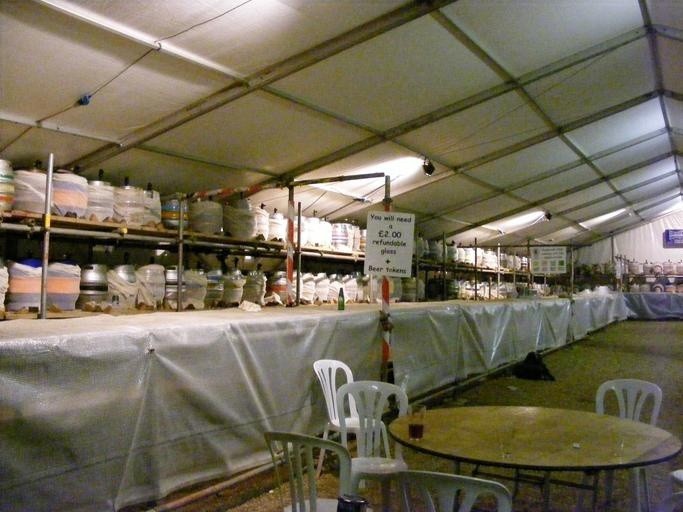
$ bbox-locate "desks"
[386,405,682,511]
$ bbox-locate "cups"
[408,404,427,442]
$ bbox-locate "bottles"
[337,287,345,310]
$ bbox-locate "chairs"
[398,463,513,512]
[315,359,392,494]
[263,431,352,512]
[334,380,409,510]
[574,378,663,512]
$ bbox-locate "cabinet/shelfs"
[530,248,683,293]
[0,153,529,319]
